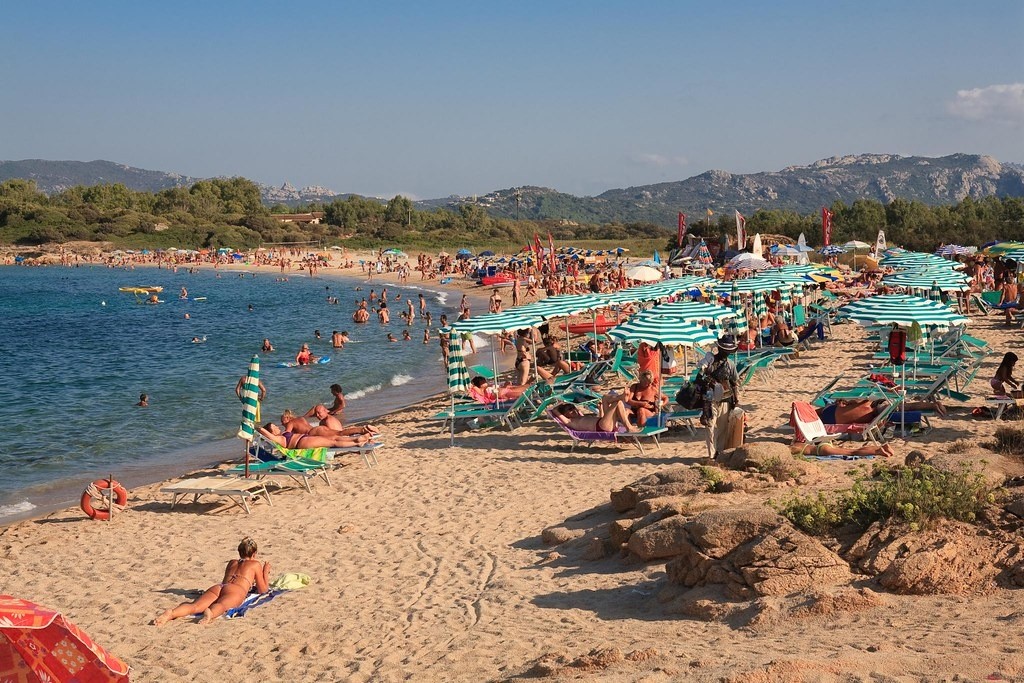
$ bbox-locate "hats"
[714,334,739,352]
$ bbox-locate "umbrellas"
[0,595,133,683]
[237,354,260,478]
[447,326,471,447]
[115,238,1024,443]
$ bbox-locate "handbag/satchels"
[674,381,706,410]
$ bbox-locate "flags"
[707,209,713,216]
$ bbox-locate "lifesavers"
[80,478,128,520]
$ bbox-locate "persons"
[4,242,1024,457]
[152,536,271,626]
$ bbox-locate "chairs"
[430,290,1024,454]
[160,430,384,514]
